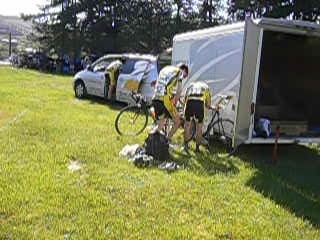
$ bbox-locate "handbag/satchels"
[254,116,273,138]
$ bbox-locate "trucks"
[158,15,320,151]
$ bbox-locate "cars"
[72,53,158,106]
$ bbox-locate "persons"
[182,81,212,151]
[152,65,188,140]
[104,58,126,104]
[9,46,95,76]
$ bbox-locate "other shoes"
[181,147,188,150]
[195,149,200,152]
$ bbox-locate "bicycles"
[115,88,239,159]
